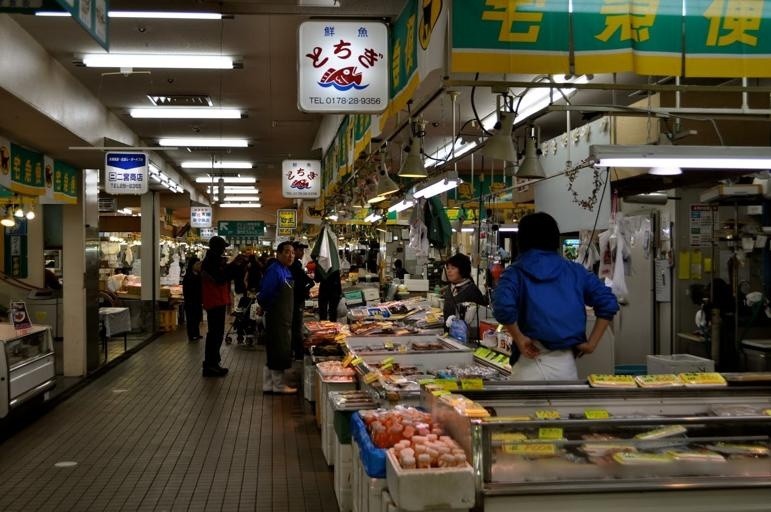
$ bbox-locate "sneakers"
[203,366,228,377]
[189,337,198,341]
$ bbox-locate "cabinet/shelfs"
[709,198,771,349]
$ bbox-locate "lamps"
[516,122,546,178]
[148,160,262,208]
[71,54,244,70]
[35,10,235,20]
[351,90,464,223]
[152,137,255,147]
[649,132,682,176]
[590,144,770,170]
[0,194,38,227]
[478,92,517,162]
[120,106,248,119]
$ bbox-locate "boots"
[263,366,297,393]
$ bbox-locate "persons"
[394,259,410,279]
[114,265,130,276]
[491,210,620,381]
[182,239,350,395]
[443,252,490,344]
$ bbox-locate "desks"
[98,307,132,363]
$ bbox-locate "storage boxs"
[305,356,475,512]
[646,353,715,374]
[742,339,771,371]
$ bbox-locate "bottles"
[451,317,465,342]
[404,274,410,285]
[434,285,440,294]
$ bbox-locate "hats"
[209,236,229,250]
